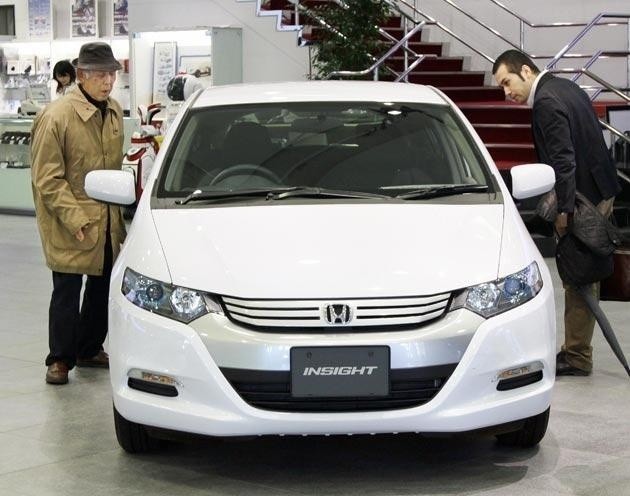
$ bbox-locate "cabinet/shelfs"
[0,113,38,217]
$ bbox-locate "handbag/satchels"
[555,233,616,289]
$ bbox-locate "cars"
[83,79,558,452]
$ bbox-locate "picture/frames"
[179,54,211,81]
[26,0,53,40]
[69,0,99,39]
[111,0,128,36]
[151,41,177,109]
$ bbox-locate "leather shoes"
[45,361,68,384]
[556,358,589,377]
[75,350,109,367]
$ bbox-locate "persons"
[29,39,126,385]
[492,48,618,377]
[52,60,77,98]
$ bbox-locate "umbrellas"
[553,224,630,377]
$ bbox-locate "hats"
[71,41,123,71]
